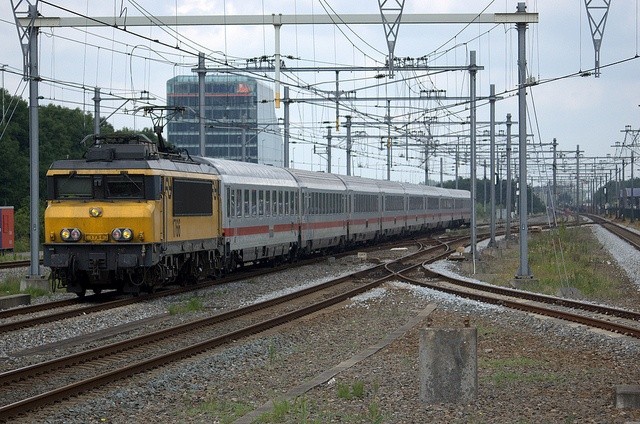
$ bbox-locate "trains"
[44,135,472,298]
